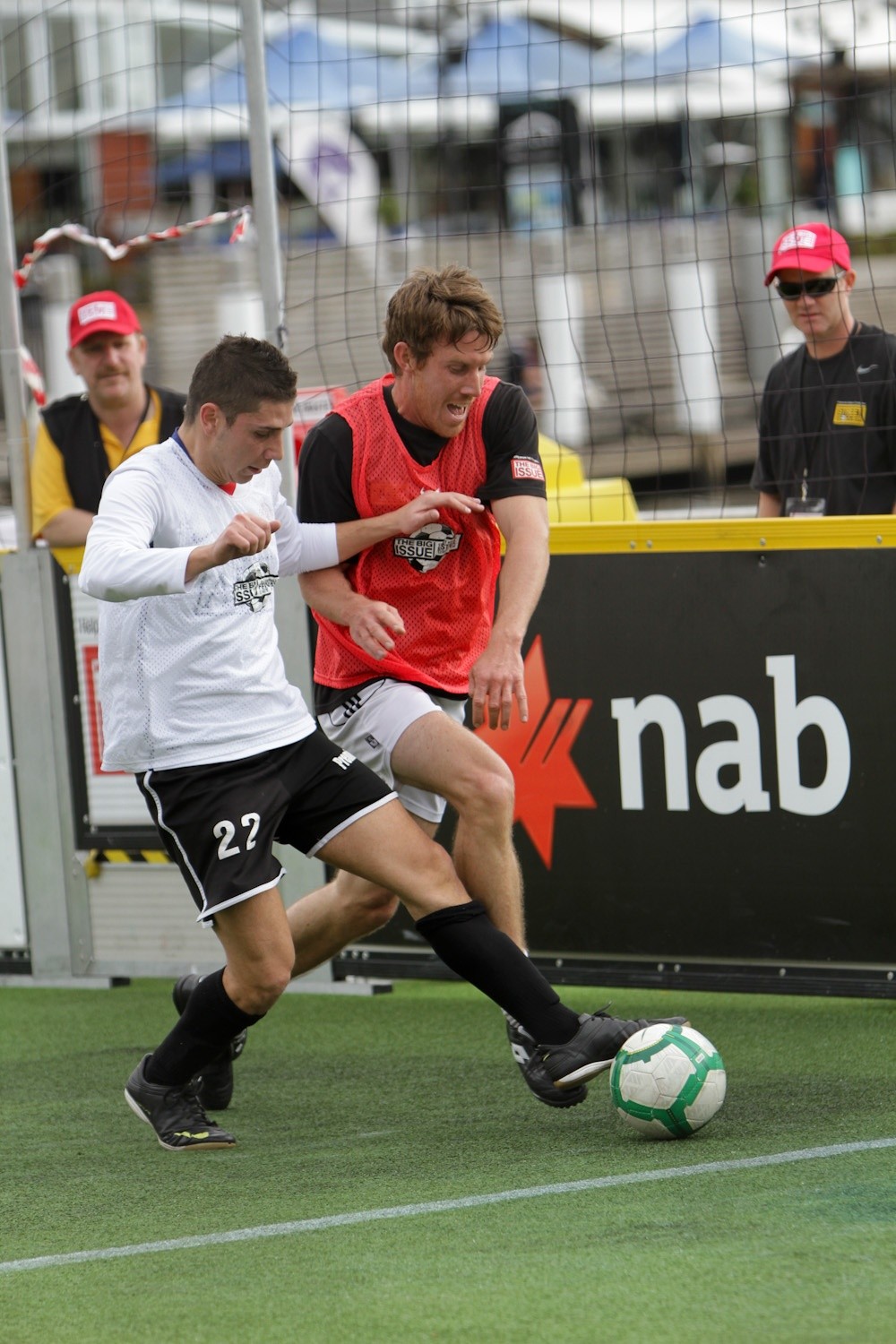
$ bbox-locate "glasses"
[769,270,849,301]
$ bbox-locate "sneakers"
[539,1005,693,1097]
[500,1012,586,1110]
[172,969,241,1112]
[121,1054,240,1157]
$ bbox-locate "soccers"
[607,1021,729,1140]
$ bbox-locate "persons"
[30,290,191,579]
[173,263,592,1108]
[748,223,896,518]
[77,334,689,1158]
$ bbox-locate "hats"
[762,221,854,288]
[68,287,143,348]
